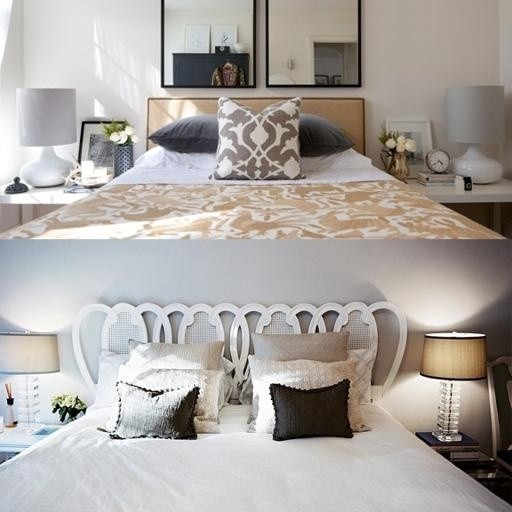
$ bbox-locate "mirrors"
[161,0,256,88]
[265,1,364,88]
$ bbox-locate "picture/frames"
[385,119,434,178]
[186,24,209,54]
[77,121,125,187]
[486,356,512,470]
[211,23,238,53]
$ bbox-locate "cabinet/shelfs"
[173,53,250,86]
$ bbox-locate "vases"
[389,152,409,180]
[114,144,132,176]
[67,417,77,425]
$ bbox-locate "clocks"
[426,148,450,174]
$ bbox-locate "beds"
[3,98,511,239]
[0,304,510,511]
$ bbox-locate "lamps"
[22,88,77,188]
[420,333,486,442]
[444,86,504,184]
[2,332,59,436]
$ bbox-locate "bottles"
[5,398,18,428]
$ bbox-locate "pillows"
[250,331,350,363]
[96,352,130,420]
[248,355,369,433]
[128,369,223,434]
[209,97,306,180]
[349,349,374,403]
[299,112,355,156]
[111,382,199,440]
[270,378,353,440]
[124,339,224,370]
[148,113,217,153]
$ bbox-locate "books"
[416,170,457,187]
[416,430,496,469]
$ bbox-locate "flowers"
[379,128,416,153]
[103,119,134,145]
[53,395,86,421]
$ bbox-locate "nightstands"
[0,179,101,226]
[383,166,511,234]
[0,422,62,464]
[426,449,512,489]
